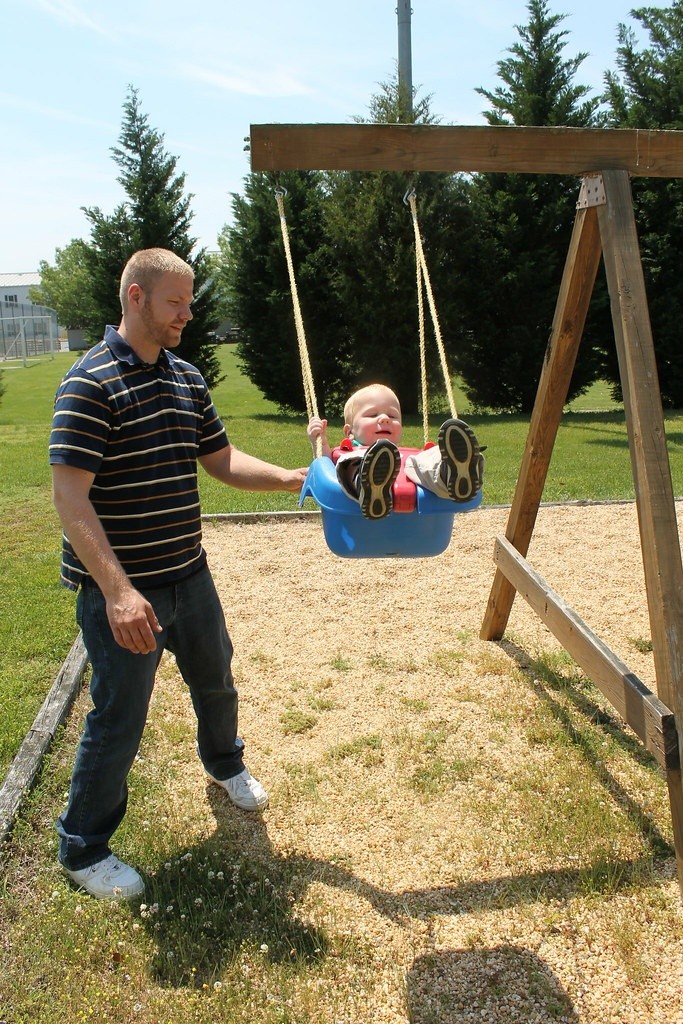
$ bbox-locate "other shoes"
[438,418,487,502]
[356,439,403,521]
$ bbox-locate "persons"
[48,248,308,899]
[308,383,488,520]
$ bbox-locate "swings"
[270,167,485,560]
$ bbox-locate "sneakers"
[62,853,144,900]
[204,766,269,810]
